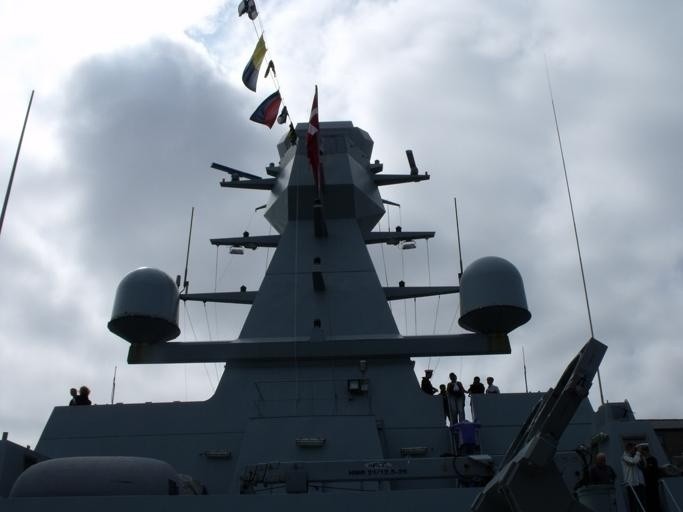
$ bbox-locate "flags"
[307,85,325,192]
[242,38,276,91]
[277,124,297,158]
[249,92,288,128]
[237,0,259,21]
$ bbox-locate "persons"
[439,385,450,421]
[589,452,616,486]
[421,370,438,395]
[470,377,485,392]
[68,387,91,407]
[638,443,661,512]
[447,373,469,423]
[620,442,648,512]
[486,377,499,395]
